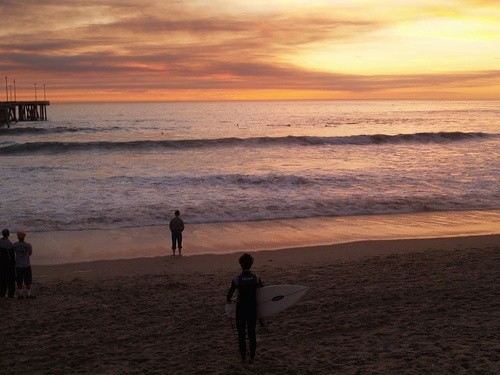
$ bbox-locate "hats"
[17,232,26,239]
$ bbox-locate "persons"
[226,253,264,365]
[169,210,185,257]
[0,228,16,300]
[12,231,38,300]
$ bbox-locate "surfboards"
[223,284,311,321]
